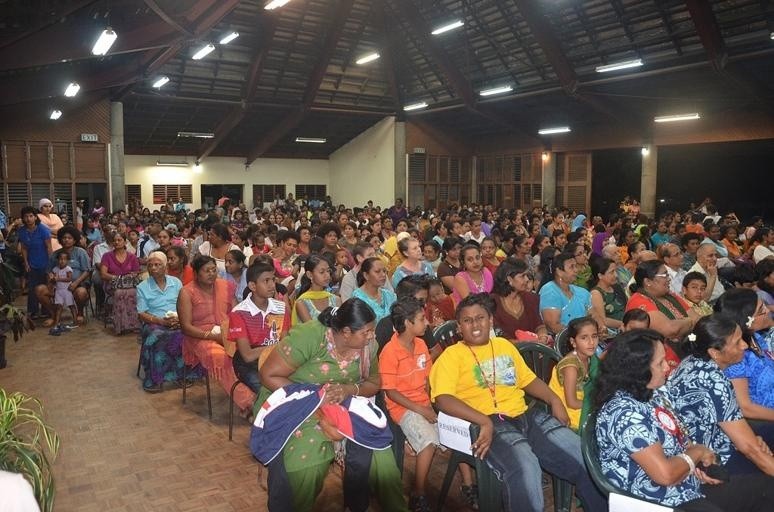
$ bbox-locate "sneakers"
[40,313,48,317]
[31,312,39,319]
[459,481,481,512]
[409,491,434,511]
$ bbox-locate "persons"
[378,298,476,511]
[486,259,546,348]
[546,316,600,432]
[0,194,773,411]
[714,288,774,421]
[427,296,608,511]
[591,330,773,511]
[251,298,411,511]
[537,252,593,333]
[668,315,774,484]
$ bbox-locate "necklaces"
[647,394,698,447]
[463,340,504,421]
[747,334,761,356]
[573,351,589,379]
[501,292,524,317]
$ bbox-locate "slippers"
[43,318,56,327]
[144,384,160,391]
[77,316,83,325]
[239,412,254,425]
[174,378,192,388]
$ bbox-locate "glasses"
[752,296,766,318]
[655,272,669,278]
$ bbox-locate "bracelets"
[674,452,696,476]
[352,381,363,399]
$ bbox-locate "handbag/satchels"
[111,272,141,289]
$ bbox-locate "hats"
[39,199,53,212]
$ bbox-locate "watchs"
[620,310,648,329]
